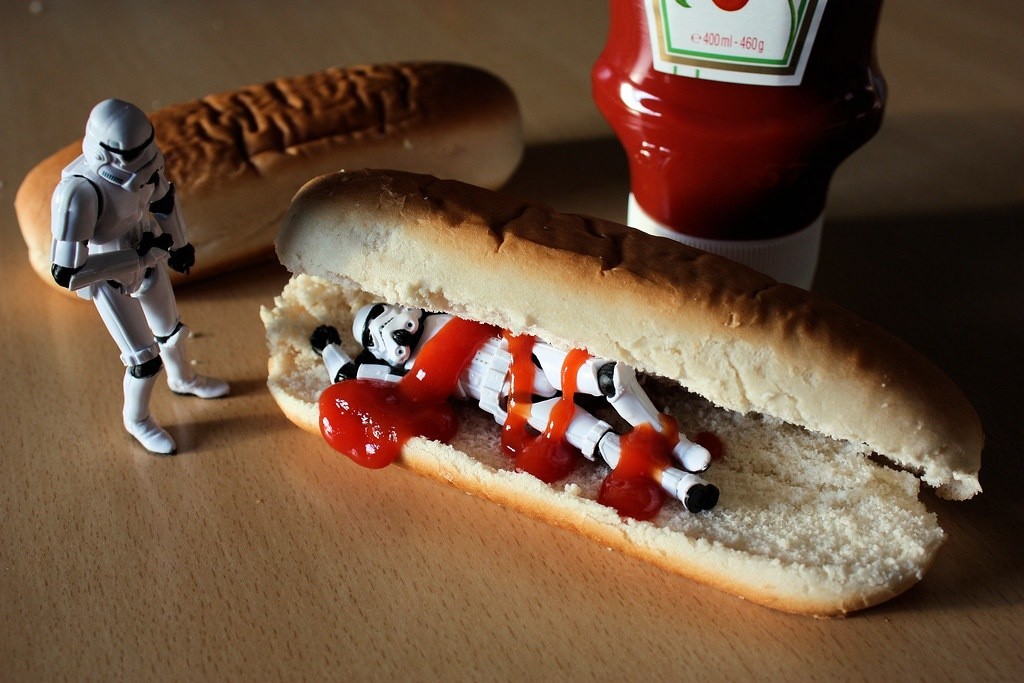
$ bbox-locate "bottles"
[590,0,889,289]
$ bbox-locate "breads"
[13,60,523,296]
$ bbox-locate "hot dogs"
[259,169,987,618]
